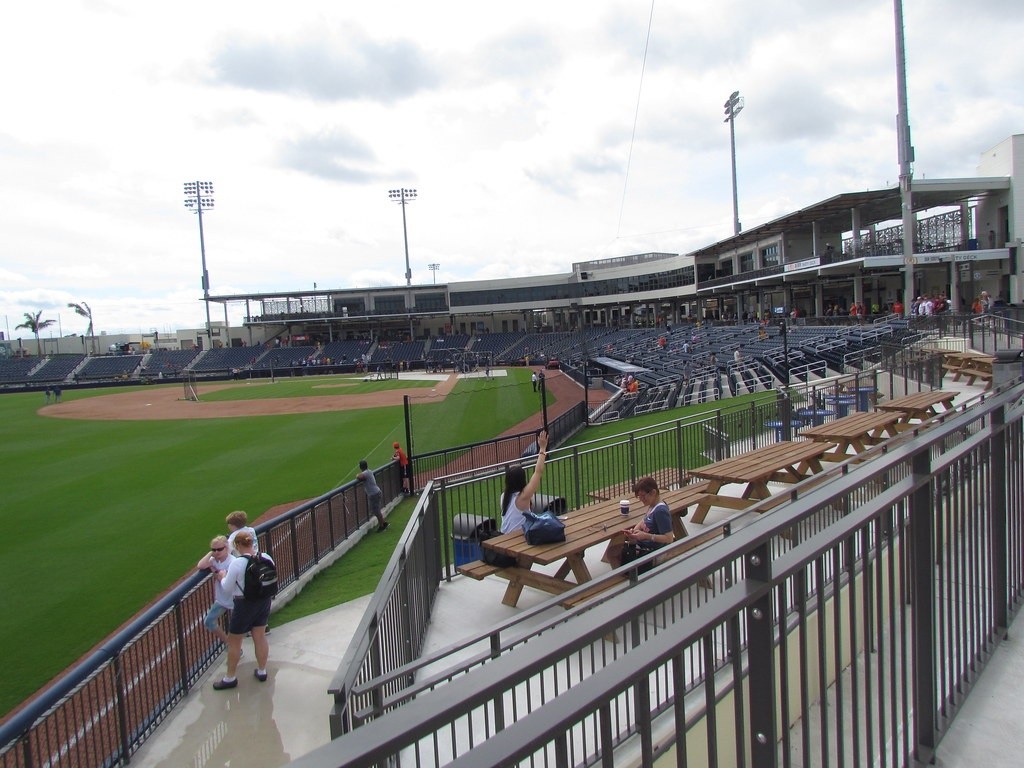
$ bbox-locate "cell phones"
[623,530,632,534]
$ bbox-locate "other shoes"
[375,526,386,533]
[226,649,244,664]
[247,624,271,636]
[255,668,267,681]
[383,522,390,528]
[213,677,238,690]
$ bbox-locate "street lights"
[387,187,417,342]
[723,89,744,235]
[427,263,439,286]
[184,179,214,347]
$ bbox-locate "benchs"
[908,358,994,380]
[455,472,847,619]
[585,467,694,501]
[0,316,909,422]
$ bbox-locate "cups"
[620,500,631,516]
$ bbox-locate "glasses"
[637,492,652,500]
[211,546,226,552]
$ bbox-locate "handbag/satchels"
[522,509,566,546]
[479,530,516,567]
[621,543,653,576]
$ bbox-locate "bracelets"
[651,534,655,543]
[540,451,546,455]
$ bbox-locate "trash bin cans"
[530,494,567,516]
[450,513,497,566]
[992,349,1023,393]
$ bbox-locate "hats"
[393,442,399,447]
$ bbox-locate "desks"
[799,411,910,487]
[824,394,853,402]
[848,386,875,413]
[826,399,856,420]
[763,419,802,443]
[686,439,849,541]
[798,410,833,425]
[914,348,994,390]
[481,485,715,646]
[869,390,969,451]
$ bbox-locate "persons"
[615,373,638,393]
[524,354,529,366]
[911,293,949,322]
[532,372,537,392]
[398,359,410,371]
[608,342,613,352]
[212,531,275,690]
[501,431,550,535]
[356,460,391,533]
[219,340,260,348]
[892,300,904,318]
[972,290,991,313]
[224,511,270,635]
[232,367,240,380]
[537,369,545,390]
[623,477,673,575]
[655,308,771,382]
[391,442,409,494]
[823,303,846,325]
[849,302,864,321]
[871,301,882,314]
[287,356,331,366]
[825,243,833,253]
[45,385,62,404]
[790,307,799,325]
[197,536,244,664]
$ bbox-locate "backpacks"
[235,551,278,605]
